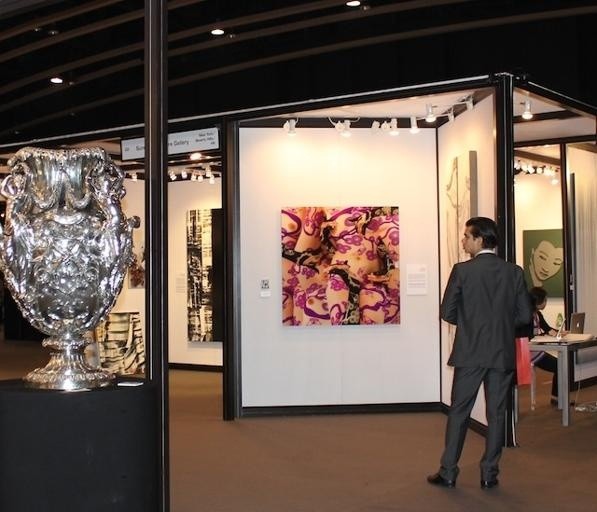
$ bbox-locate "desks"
[514,339,595,428]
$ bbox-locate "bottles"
[556,312,564,329]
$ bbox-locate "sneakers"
[551,397,575,406]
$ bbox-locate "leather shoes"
[427,472,455,488]
[481,479,498,489]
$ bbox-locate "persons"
[281,206,400,324]
[526,287,577,408]
[426,216,535,488]
[523,240,564,291]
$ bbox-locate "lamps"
[282,97,475,138]
[168,165,215,185]
[130,170,136,181]
[520,96,533,121]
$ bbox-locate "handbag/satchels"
[514,336,533,386]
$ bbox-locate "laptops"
[530,318,566,343]
[570,312,585,334]
